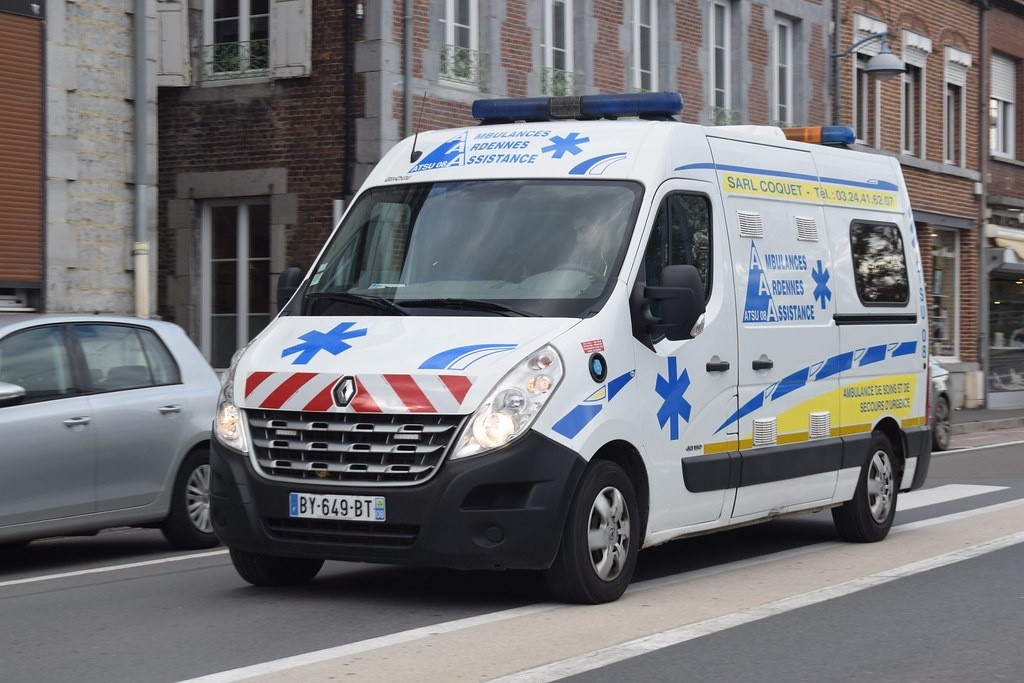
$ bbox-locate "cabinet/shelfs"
[932,249,956,357]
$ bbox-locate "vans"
[207,94,935,599]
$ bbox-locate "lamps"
[827,33,907,96]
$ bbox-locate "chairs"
[88,365,148,387]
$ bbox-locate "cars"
[928,354,955,451]
[0,310,222,547]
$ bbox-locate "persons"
[571,202,623,286]
